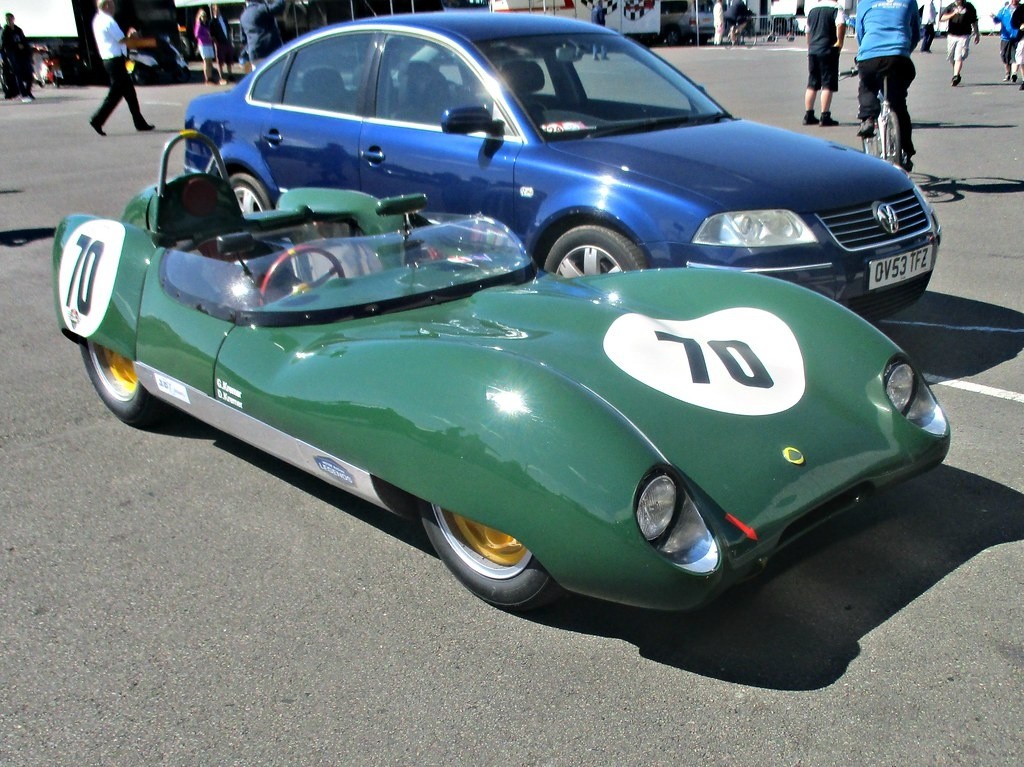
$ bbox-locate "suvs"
[660,0,715,46]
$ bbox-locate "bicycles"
[840,69,902,167]
[721,13,757,49]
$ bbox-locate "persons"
[723,0,752,45]
[0,13,34,103]
[591,0,609,60]
[712,0,725,45]
[239,0,282,71]
[803,0,846,126]
[391,61,459,127]
[920,0,936,53]
[194,5,235,86]
[939,0,980,86]
[856,0,920,173]
[990,0,1024,91]
[89,0,155,136]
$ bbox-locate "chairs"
[156,130,291,308]
[504,61,548,126]
[390,59,451,126]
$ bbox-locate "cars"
[54,129,952,613]
[183,10,941,319]
[845,12,855,35]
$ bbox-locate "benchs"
[301,63,397,108]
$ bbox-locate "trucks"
[28,0,183,84]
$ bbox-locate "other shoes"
[1011,73,1018,83]
[1018,81,1024,90]
[1003,74,1010,82]
[857,116,875,136]
[951,74,961,85]
[903,155,913,172]
[819,111,839,127]
[803,110,820,125]
[138,125,155,131]
[89,120,107,136]
